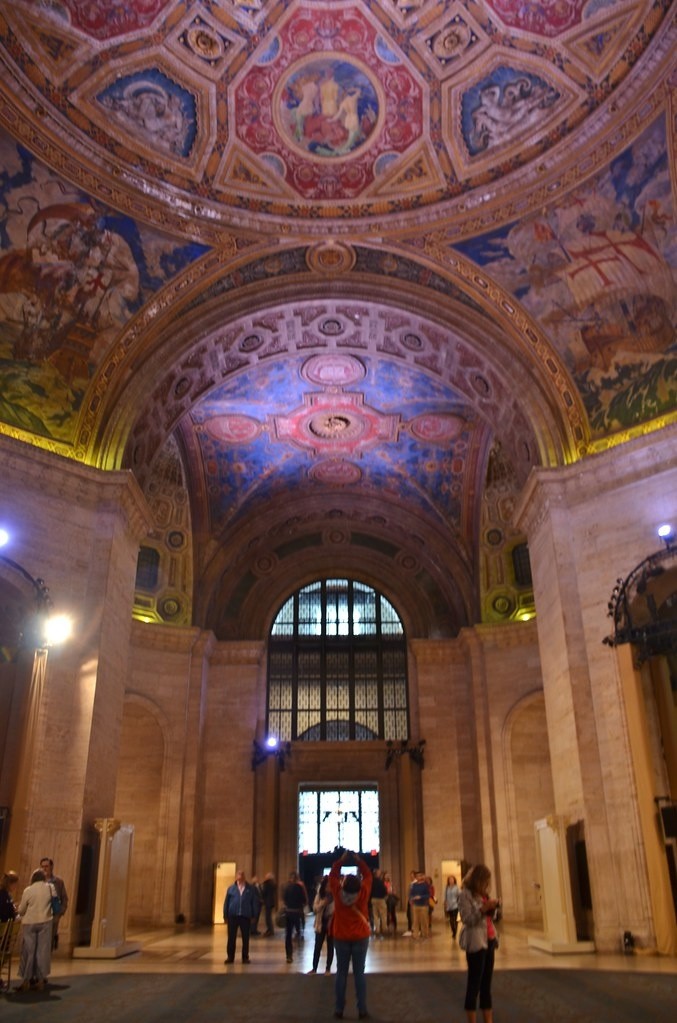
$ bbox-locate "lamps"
[384,735,426,771]
[658,525,674,549]
[253,736,291,771]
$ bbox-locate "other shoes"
[224,959,234,964]
[358,1012,368,1018]
[263,930,273,936]
[242,960,250,963]
[286,958,293,963]
[34,982,44,992]
[22,979,31,991]
[402,930,412,936]
[334,1011,343,1019]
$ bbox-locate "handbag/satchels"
[49,885,61,916]
[428,896,438,908]
[459,925,469,950]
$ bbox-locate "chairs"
[0,918,21,993]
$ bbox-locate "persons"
[458,862,503,1023]
[0,857,68,993]
[443,874,460,940]
[340,869,396,933]
[402,870,438,939]
[280,872,307,963]
[307,875,335,976]
[328,852,373,1019]
[223,870,277,964]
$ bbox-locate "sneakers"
[324,971,330,977]
[307,971,317,976]
